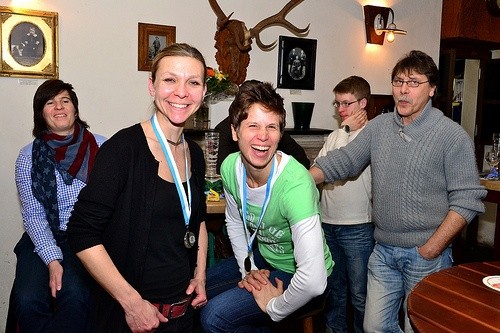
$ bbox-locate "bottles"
[202,131,223,202]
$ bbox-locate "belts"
[152,294,194,319]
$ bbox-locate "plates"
[482,275,500,292]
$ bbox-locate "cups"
[291,101,315,133]
[493,133,500,152]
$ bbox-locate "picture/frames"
[276,34,321,90]
[137,22,178,71]
[0,6,60,80]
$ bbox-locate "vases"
[291,102,317,128]
[193,105,212,129]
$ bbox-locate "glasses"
[333,98,363,107]
[391,80,430,87]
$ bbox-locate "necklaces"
[167,138,181,145]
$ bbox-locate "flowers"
[204,67,237,105]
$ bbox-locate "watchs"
[339,125,350,134]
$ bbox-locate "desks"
[206,196,226,265]
[408,262,500,333]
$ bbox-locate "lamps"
[361,5,408,46]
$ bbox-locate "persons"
[289,48,296,72]
[16,33,39,57]
[299,50,305,75]
[152,36,161,58]
[13,41,375,333]
[307,50,486,333]
[291,55,302,78]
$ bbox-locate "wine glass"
[484,151,500,181]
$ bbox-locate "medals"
[244,256,252,274]
[184,231,195,249]
